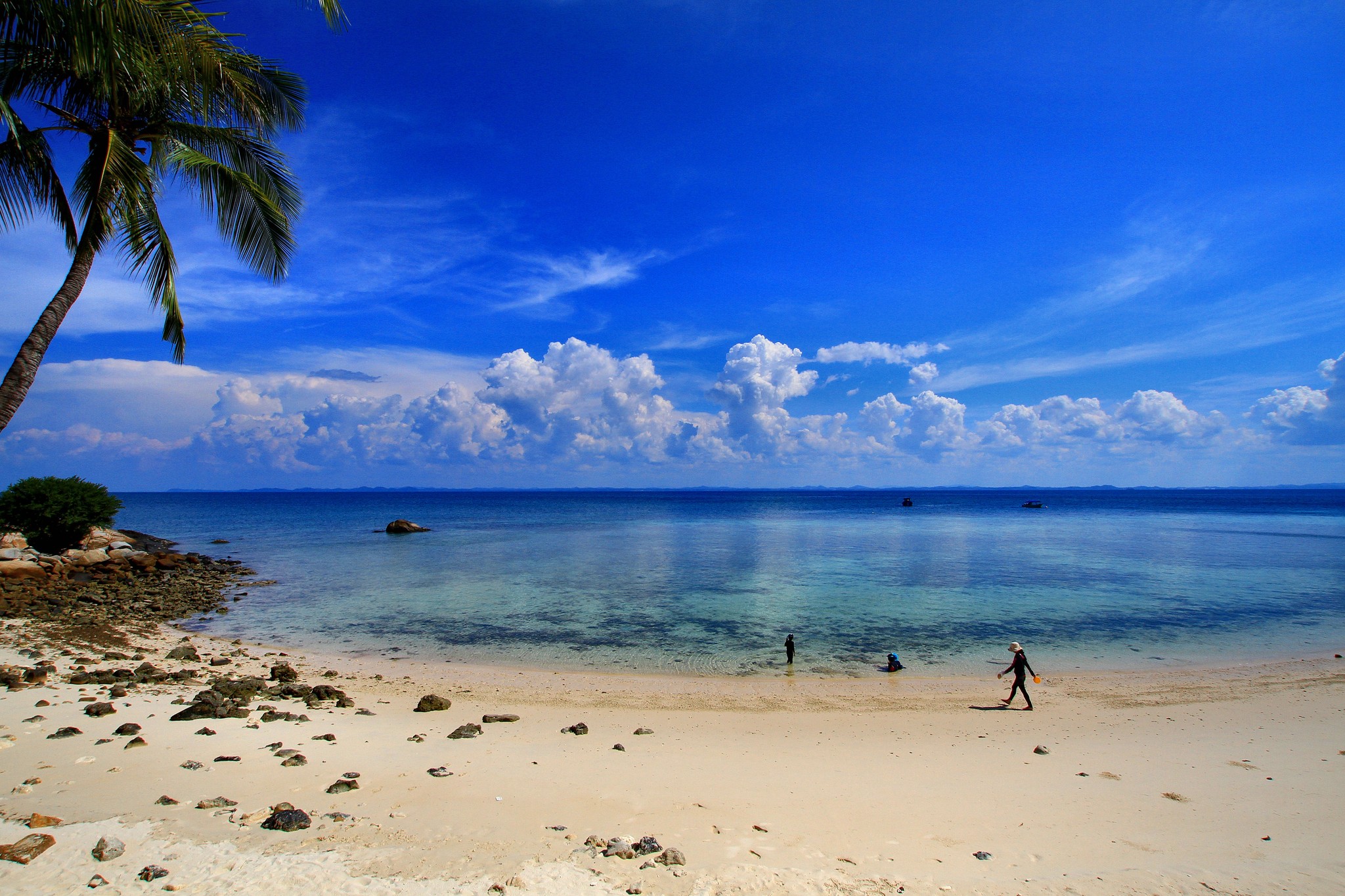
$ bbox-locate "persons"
[873,653,906,672]
[998,642,1037,710]
[784,634,795,664]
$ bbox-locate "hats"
[1008,642,1023,651]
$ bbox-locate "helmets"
[788,634,794,639]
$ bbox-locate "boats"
[1022,500,1044,508]
[902,497,912,507]
